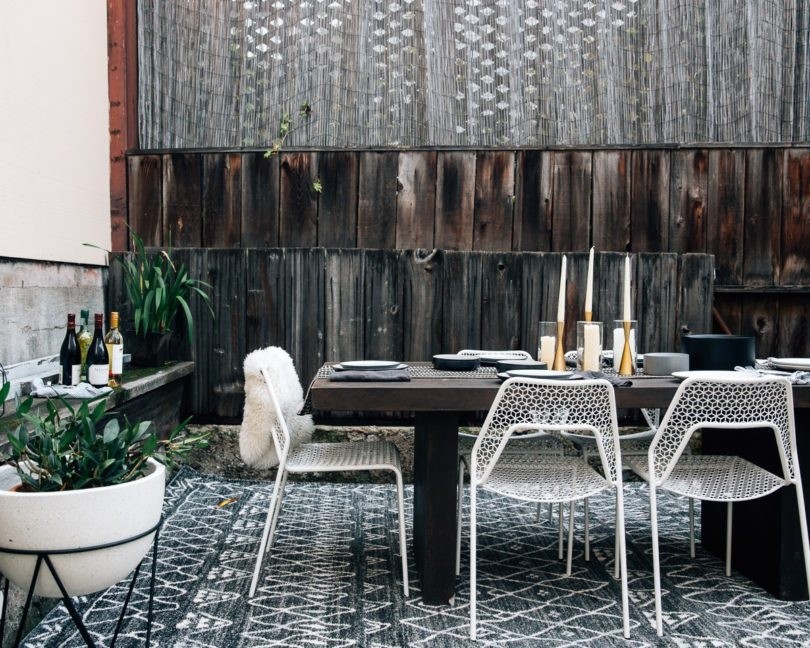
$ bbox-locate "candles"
[584,245,595,312]
[557,255,567,321]
[613,327,636,370]
[541,335,554,369]
[583,324,599,371]
[623,252,631,321]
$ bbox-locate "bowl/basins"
[433,353,481,370]
[644,353,689,376]
[681,334,756,370]
[496,360,548,372]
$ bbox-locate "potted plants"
[0,381,214,597]
[82,222,218,368]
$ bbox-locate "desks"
[311,359,810,602]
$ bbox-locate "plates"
[672,370,794,379]
[769,358,810,370]
[332,359,409,372]
[496,369,584,382]
[475,354,528,364]
[605,353,643,364]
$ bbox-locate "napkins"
[329,370,410,381]
[577,372,633,387]
[735,365,810,383]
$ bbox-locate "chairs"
[454,346,566,561]
[456,370,631,642]
[565,349,696,563]
[248,346,410,598]
[615,374,810,635]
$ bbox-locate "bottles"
[58,312,81,386]
[105,311,123,383]
[86,313,110,388]
[77,308,94,383]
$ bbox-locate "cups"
[537,321,559,369]
[577,321,604,371]
[614,320,638,374]
